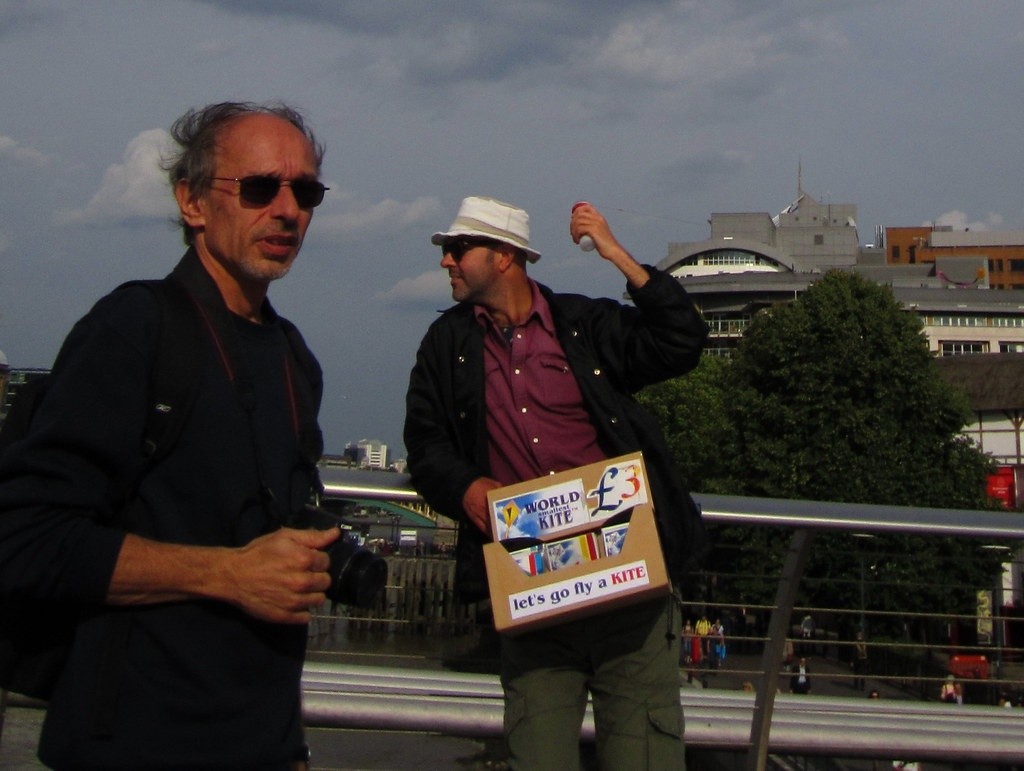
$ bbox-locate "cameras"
[288,504,389,612]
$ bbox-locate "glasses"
[443,239,498,260]
[206,175,330,208]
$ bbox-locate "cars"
[924,648,991,680]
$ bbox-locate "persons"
[783,610,816,695]
[1,103,341,771]
[848,632,872,692]
[681,615,729,667]
[998,689,1023,707]
[940,673,962,704]
[404,195,709,771]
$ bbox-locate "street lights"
[851,533,876,632]
[980,544,1010,679]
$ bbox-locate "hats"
[432,197,543,263]
